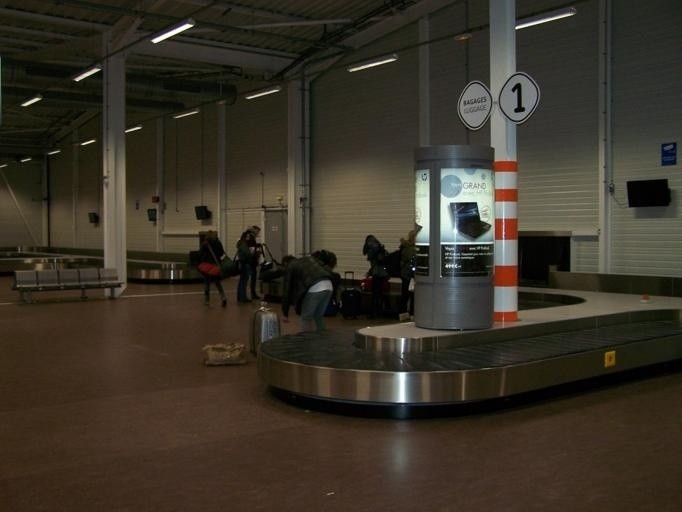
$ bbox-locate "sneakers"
[221,296,227,307]
[237,293,260,303]
[204,300,210,305]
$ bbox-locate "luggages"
[341,271,360,320]
[249,305,281,355]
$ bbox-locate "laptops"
[450,202,492,238]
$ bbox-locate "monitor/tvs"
[195,205,208,220]
[626,179,671,207]
[88,213,97,223]
[147,209,157,221]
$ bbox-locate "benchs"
[11,268,125,304]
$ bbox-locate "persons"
[280,255,333,333]
[237,225,262,305]
[362,235,393,323]
[198,229,230,305]
[398,230,416,323]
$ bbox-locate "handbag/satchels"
[196,262,220,278]
[220,256,239,279]
[259,260,283,282]
[311,250,337,268]
[202,341,247,365]
